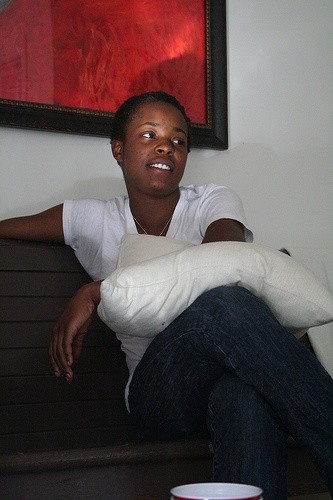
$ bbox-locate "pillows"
[97,241,333,338]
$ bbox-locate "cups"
[169,482,264,500]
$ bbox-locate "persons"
[0,90,333,500]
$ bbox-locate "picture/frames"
[1,1,229,150]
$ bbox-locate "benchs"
[0,238,333,500]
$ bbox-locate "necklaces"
[133,213,171,236]
